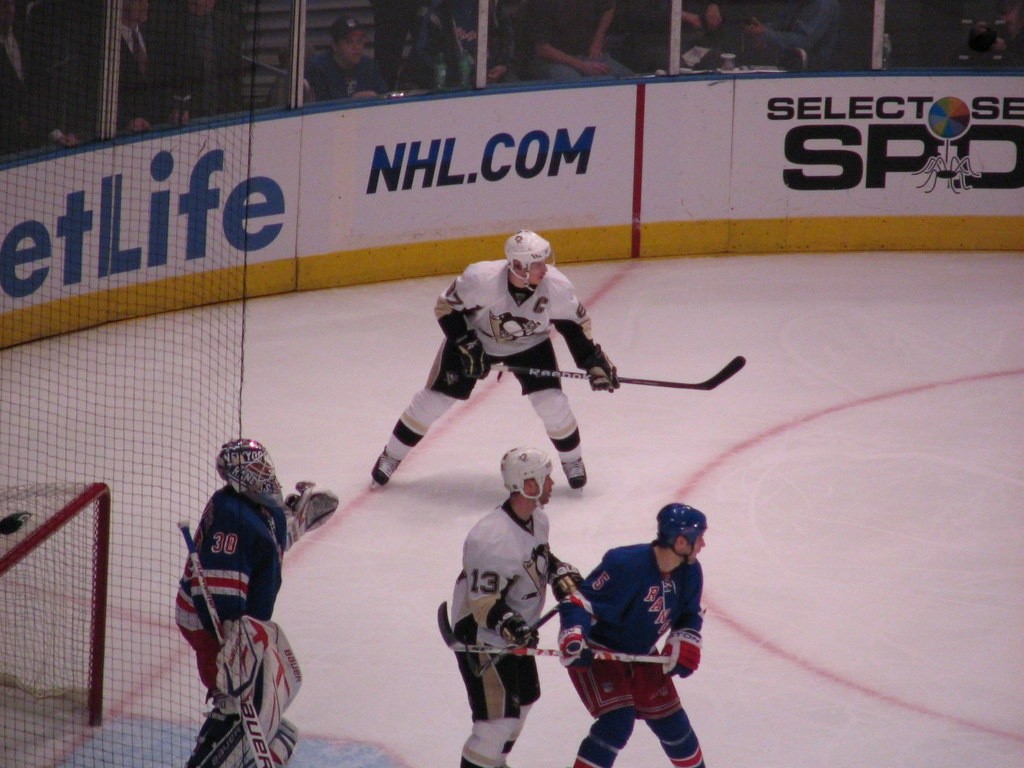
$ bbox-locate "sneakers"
[561,457,587,496]
[370,445,402,489]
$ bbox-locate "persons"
[0,0,1023,158]
[449,446,584,768]
[372,229,620,485]
[559,503,708,768]
[174,439,339,768]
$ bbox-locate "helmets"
[504,230,559,291]
[500,447,553,510]
[216,439,284,507]
[656,503,708,566]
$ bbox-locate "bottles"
[458,50,471,88]
[432,52,446,87]
[882,32,891,70]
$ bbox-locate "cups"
[719,53,736,70]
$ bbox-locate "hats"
[330,15,368,39]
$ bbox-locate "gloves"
[455,329,490,379]
[285,480,339,531]
[494,610,534,648]
[581,343,620,393]
[548,562,585,602]
[661,628,703,678]
[557,625,592,667]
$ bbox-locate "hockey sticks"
[177,518,275,768]
[464,602,561,677]
[435,601,672,666]
[484,355,746,392]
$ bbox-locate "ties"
[132,31,146,59]
